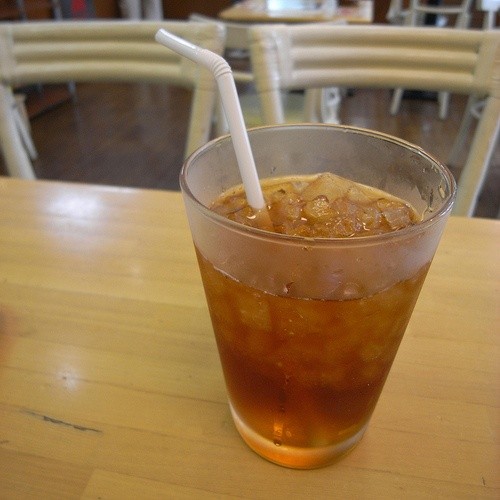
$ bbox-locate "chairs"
[248,24,499,217]
[1,21,227,183]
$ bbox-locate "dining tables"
[0,174,500,500]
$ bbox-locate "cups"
[179,120,458,471]
